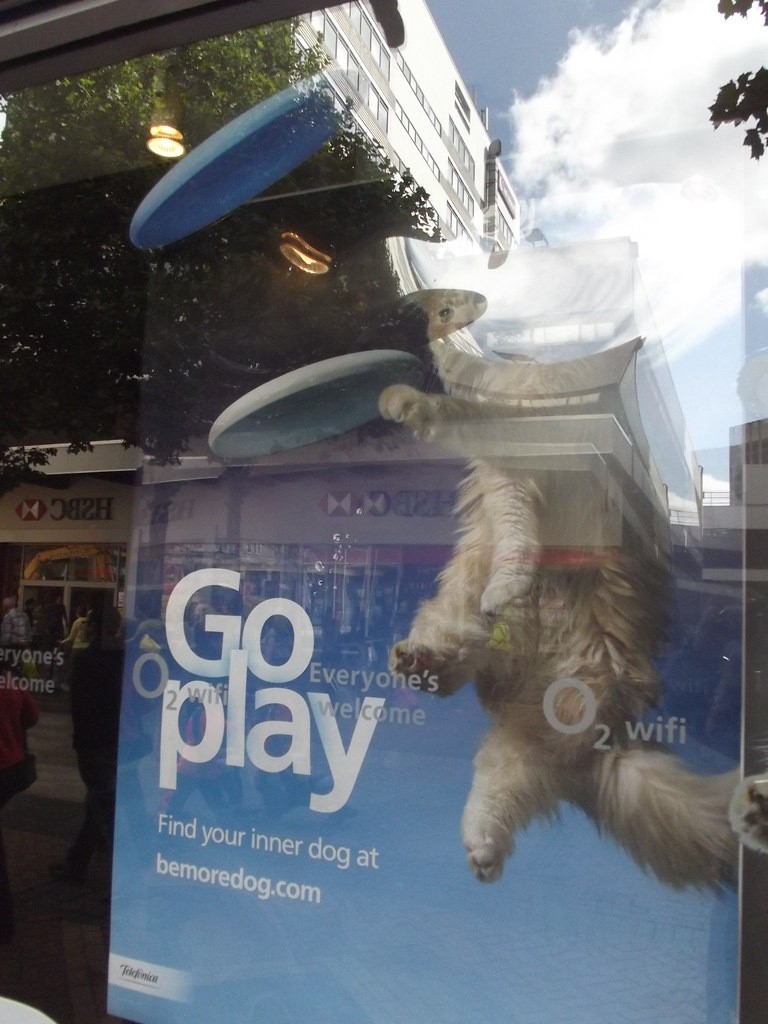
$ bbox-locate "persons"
[2,596,36,688]
[61,601,96,653]
[46,605,123,886]
[0,659,39,947]
[23,592,68,669]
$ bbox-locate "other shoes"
[46,863,87,886]
